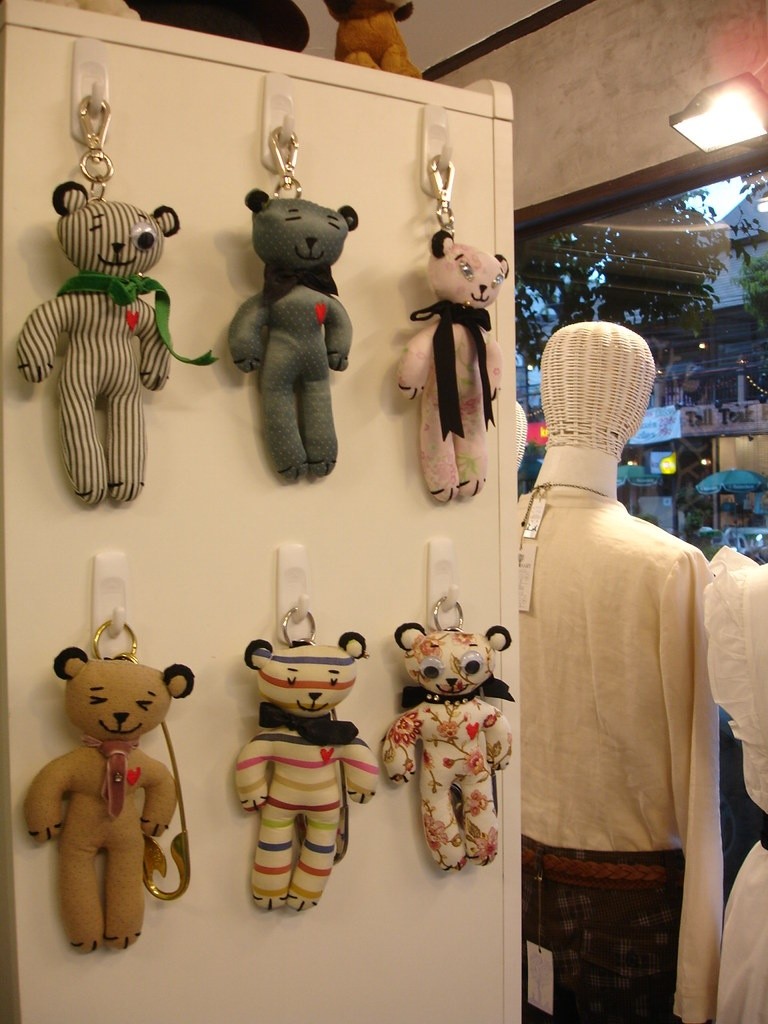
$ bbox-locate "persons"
[515,320,768,1024]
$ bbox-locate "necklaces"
[521,480,608,534]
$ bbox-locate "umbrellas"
[695,467,768,527]
[616,464,663,516]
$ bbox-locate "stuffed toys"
[233,629,383,912]
[225,187,357,482]
[393,230,510,504]
[21,644,196,956]
[16,181,181,505]
[323,0,428,81]
[379,619,516,872]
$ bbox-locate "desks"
[725,527,768,539]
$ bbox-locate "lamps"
[669,72,768,153]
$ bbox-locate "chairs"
[709,528,768,564]
